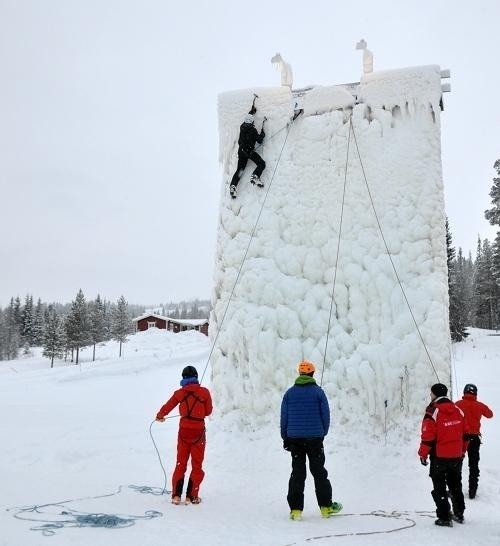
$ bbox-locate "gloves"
[283,440,291,451]
[260,129,265,138]
[251,105,256,114]
[421,458,429,466]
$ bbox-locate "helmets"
[431,383,448,397]
[244,114,255,124]
[182,366,198,379]
[298,360,315,374]
[464,384,477,396]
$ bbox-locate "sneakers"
[250,174,264,188]
[321,502,343,518]
[172,496,181,505]
[230,185,238,200]
[453,514,464,524]
[185,497,202,505]
[435,518,453,528]
[289,510,303,521]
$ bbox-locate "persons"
[153,366,214,505]
[417,383,465,527]
[230,108,266,198]
[454,385,493,498]
[279,361,342,515]
